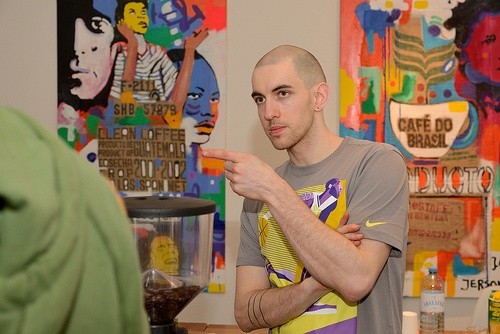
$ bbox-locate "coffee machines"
[123,195,216,328]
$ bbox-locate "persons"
[57,0,122,111]
[108,0,178,108]
[0,107,153,334]
[161,47,220,196]
[449,1,499,106]
[202,44,409,334]
[136,232,178,276]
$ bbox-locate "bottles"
[419,267,445,334]
